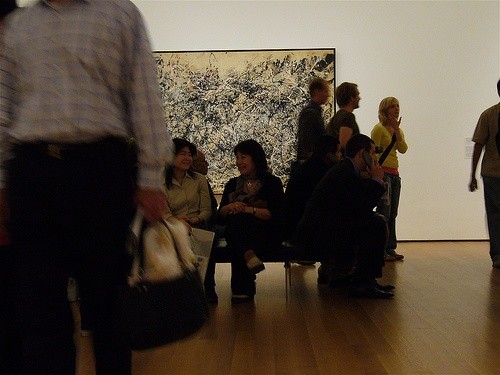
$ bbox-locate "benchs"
[216,238,300,304]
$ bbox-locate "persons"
[296,134,396,301]
[158,138,212,304]
[297,79,329,158]
[278,136,343,255]
[466,79,500,268]
[1,1,169,375]
[189,149,219,304]
[369,96,408,262]
[217,139,284,299]
[326,83,360,163]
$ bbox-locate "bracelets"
[195,215,201,221]
[252,206,256,215]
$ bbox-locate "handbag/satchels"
[108,215,208,352]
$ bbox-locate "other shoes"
[493,255,500,268]
[393,253,404,260]
[80,324,88,336]
[384,254,395,262]
[206,291,218,304]
[369,289,395,299]
[231,294,254,303]
[378,284,395,292]
[244,249,265,274]
